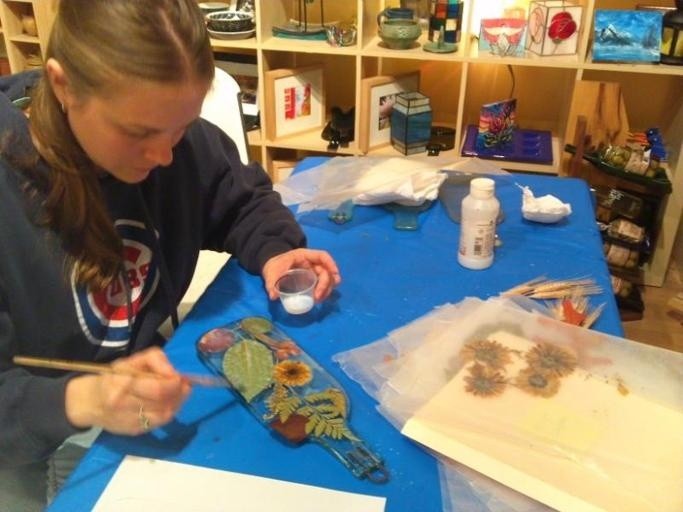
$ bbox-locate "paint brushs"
[12,355,246,394]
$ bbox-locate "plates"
[205,26,257,40]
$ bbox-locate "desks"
[46,157,621,512]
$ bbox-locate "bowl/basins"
[206,11,253,32]
[198,2,229,12]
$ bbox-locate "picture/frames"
[361,71,420,158]
[264,62,329,142]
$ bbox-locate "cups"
[273,267,318,316]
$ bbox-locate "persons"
[0,1,342,511]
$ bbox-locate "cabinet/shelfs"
[0,1,682,290]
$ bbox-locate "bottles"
[458,178,501,272]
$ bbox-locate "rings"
[134,406,153,434]
